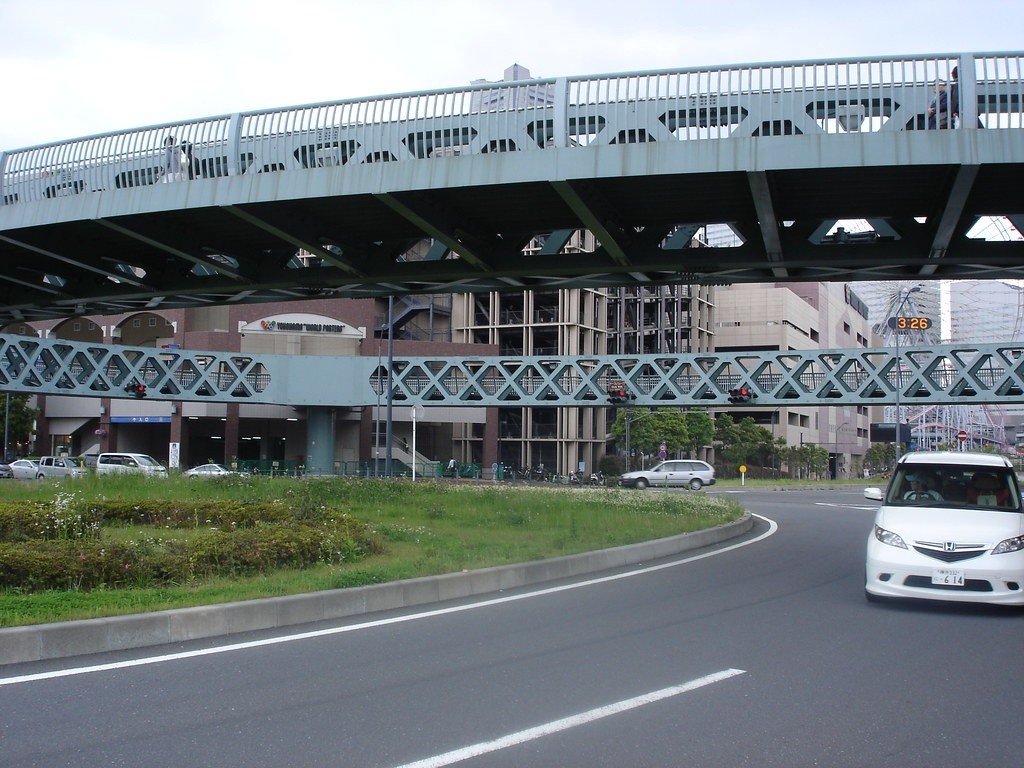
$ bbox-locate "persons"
[903,473,945,502]
[400,437,409,454]
[448,457,457,479]
[971,475,998,505]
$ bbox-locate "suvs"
[616,459,717,490]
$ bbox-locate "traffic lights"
[607,390,627,404]
[136,384,147,399]
[727,388,750,403]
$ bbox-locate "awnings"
[48,418,92,435]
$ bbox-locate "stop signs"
[958,430,966,441]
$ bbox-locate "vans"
[96,452,169,480]
[863,450,1024,609]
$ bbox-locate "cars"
[0,454,101,480]
[180,463,250,481]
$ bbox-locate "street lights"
[835,422,849,479]
[895,286,921,467]
[375,322,387,477]
